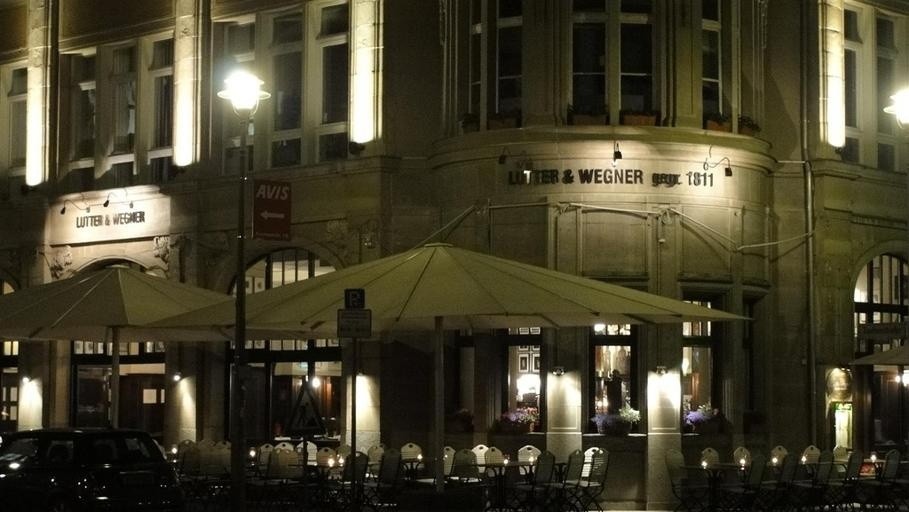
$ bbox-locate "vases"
[692,418,720,435]
[506,423,535,433]
[607,424,625,440]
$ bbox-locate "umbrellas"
[143,242,751,495]
[848,344,909,368]
[0,266,236,427]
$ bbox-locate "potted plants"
[487,110,522,130]
[448,407,489,450]
[703,109,733,133]
[567,99,609,125]
[461,111,479,134]
[738,114,762,137]
[620,109,659,126]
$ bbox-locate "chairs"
[663,441,909,511]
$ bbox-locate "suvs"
[1,427,182,509]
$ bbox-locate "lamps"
[60,199,90,214]
[498,146,534,187]
[612,138,621,174]
[102,188,133,209]
[702,156,733,178]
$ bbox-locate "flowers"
[682,400,721,426]
[589,401,642,434]
[503,404,539,426]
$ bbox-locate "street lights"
[214,67,271,509]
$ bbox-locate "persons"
[605,369,624,412]
